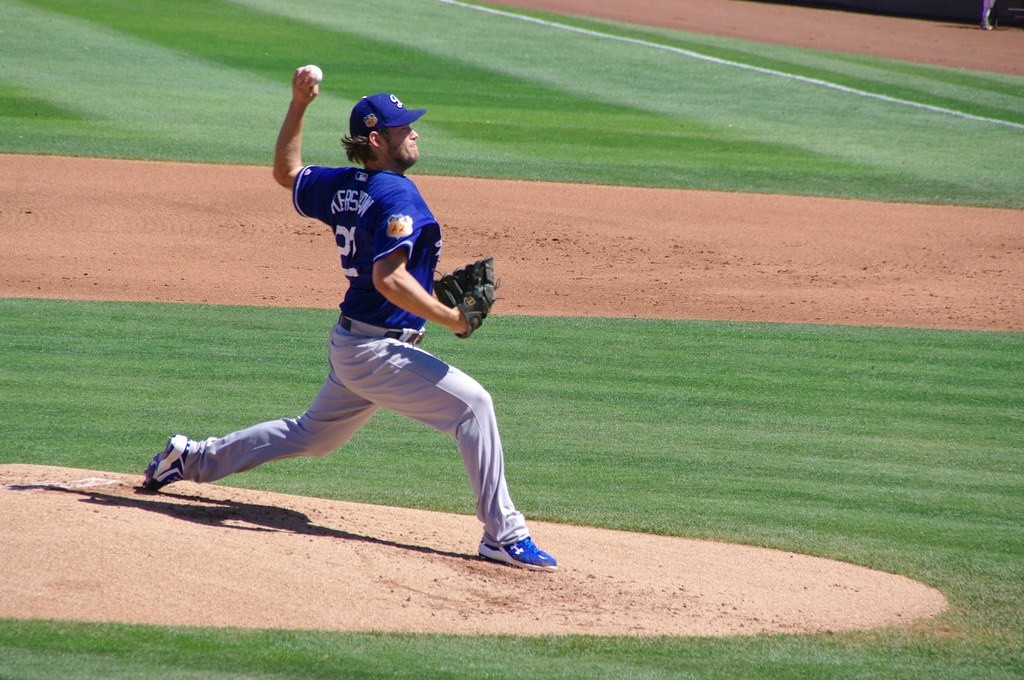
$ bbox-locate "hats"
[350,92,427,137]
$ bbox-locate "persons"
[144,68,557,571]
[980,0,995,31]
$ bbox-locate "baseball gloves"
[432,254,502,341]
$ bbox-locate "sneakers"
[478,536,557,571]
[144,434,190,492]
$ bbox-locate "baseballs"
[301,64,323,87]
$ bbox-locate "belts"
[339,314,424,345]
